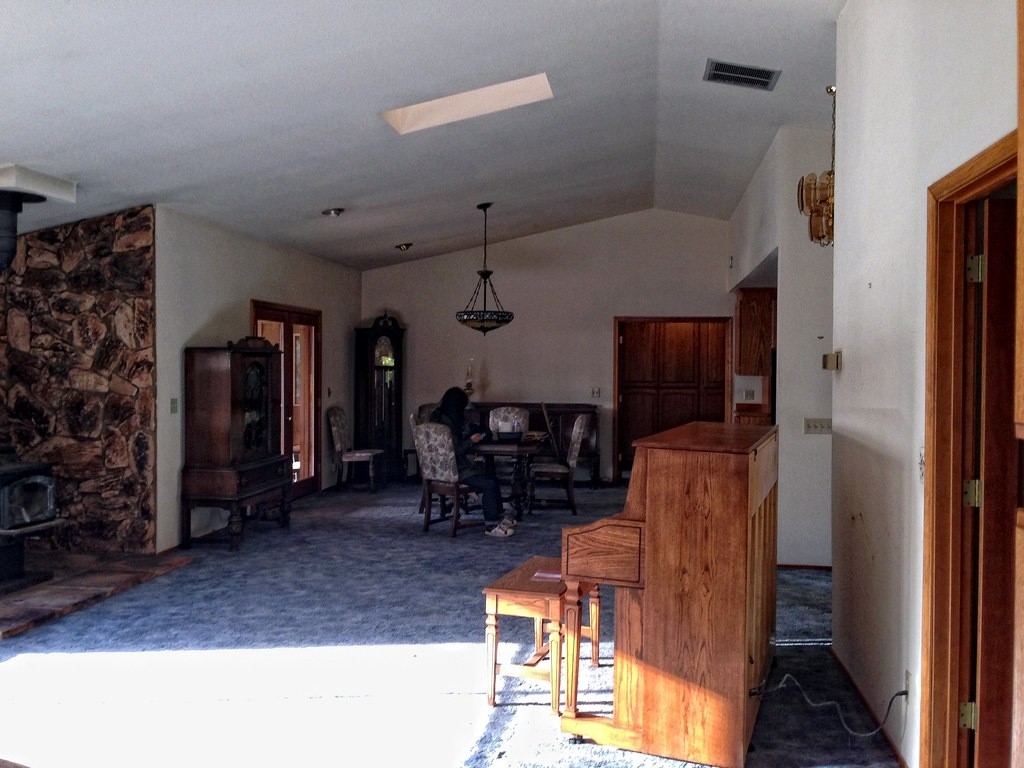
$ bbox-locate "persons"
[429,387,517,537]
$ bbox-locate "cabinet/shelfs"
[354,308,405,486]
[560,422,778,767]
[620,319,701,462]
[698,322,725,422]
[418,404,600,491]
[180,337,291,551]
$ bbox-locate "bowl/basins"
[496,431,523,442]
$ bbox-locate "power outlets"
[905,670,912,707]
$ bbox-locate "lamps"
[456,203,514,337]
[798,86,836,248]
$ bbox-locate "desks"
[465,431,547,521]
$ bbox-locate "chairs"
[412,423,474,537]
[328,406,383,494]
[409,413,469,515]
[526,414,586,517]
[487,407,529,512]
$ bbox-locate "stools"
[480,557,600,717]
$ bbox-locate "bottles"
[512,416,520,431]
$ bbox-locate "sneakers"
[485,515,517,537]
